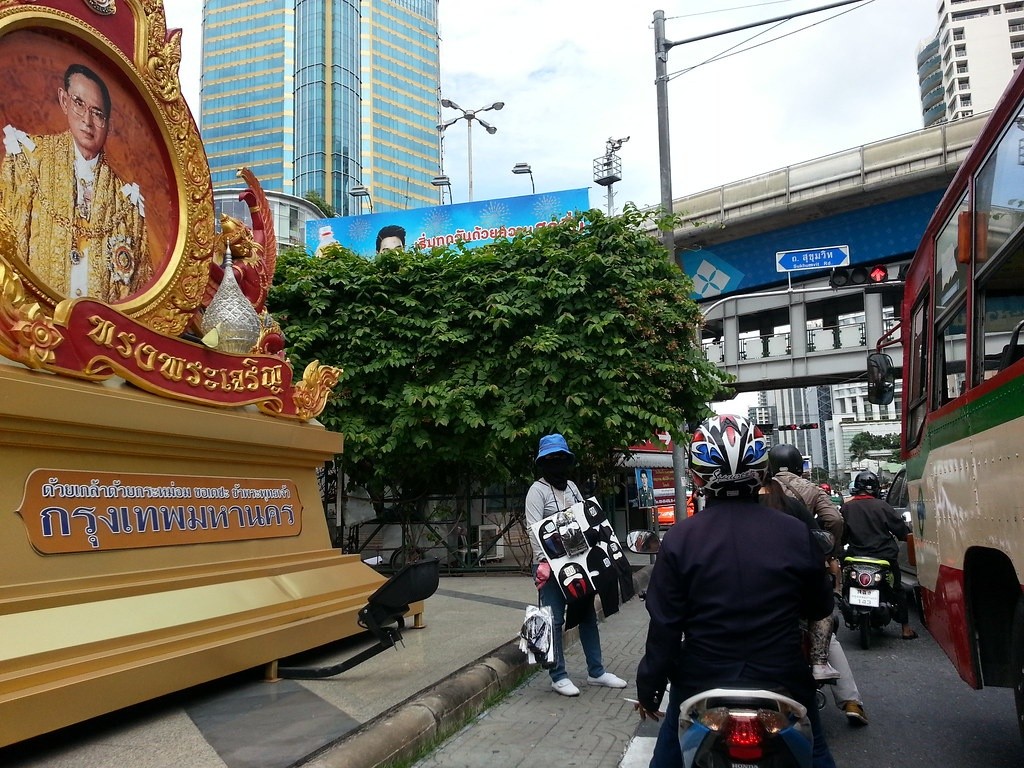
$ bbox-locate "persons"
[839,470,919,640]
[636,413,838,768]
[375,225,407,254]
[752,442,869,727]
[635,531,660,552]
[525,433,628,696]
[638,469,654,507]
[0,63,154,302]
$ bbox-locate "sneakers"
[587,672,627,688]
[551,679,578,696]
[845,700,868,725]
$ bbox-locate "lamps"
[431,175,452,204]
[512,163,535,194]
[348,185,372,214]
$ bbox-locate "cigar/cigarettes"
[623,697,640,705]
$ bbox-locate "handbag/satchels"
[519,603,555,668]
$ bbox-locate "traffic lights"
[898,262,910,280]
[800,423,818,429]
[828,265,889,287]
[778,425,797,431]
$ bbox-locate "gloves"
[535,561,551,590]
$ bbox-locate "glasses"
[66,91,109,128]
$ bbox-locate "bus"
[867,55,1023,735]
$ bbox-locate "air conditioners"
[470,523,504,563]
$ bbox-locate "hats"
[534,434,575,465]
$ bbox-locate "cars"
[883,465,923,621]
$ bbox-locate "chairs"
[457,527,481,567]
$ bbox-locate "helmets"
[688,414,770,497]
[854,470,880,497]
[819,484,831,495]
[769,443,803,475]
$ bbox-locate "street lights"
[436,100,505,203]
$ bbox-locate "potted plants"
[848,432,904,464]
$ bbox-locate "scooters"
[626,530,812,768]
[834,512,913,643]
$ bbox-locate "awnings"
[613,453,689,469]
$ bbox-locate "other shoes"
[902,630,917,639]
